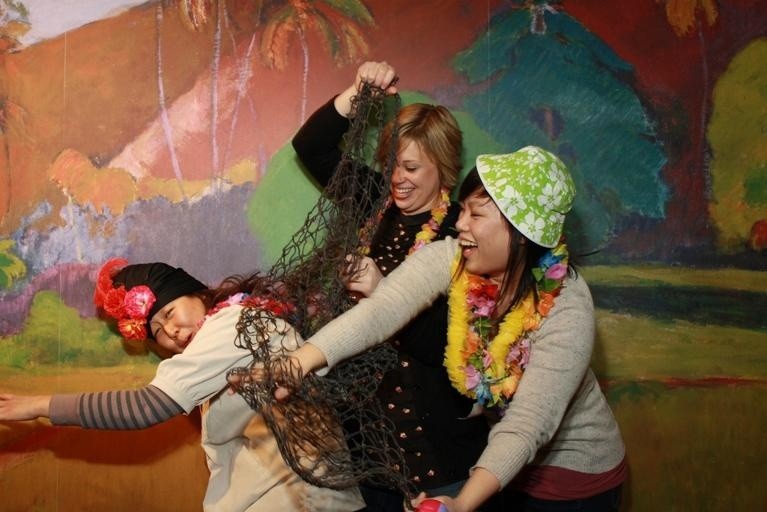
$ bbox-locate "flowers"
[189,293,295,330]
[443,239,569,408]
[93,257,156,342]
[358,184,451,261]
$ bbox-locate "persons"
[292,59,495,512]
[0,256,368,512]
[224,144,627,512]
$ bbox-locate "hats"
[476,145,575,248]
[93,258,208,342]
